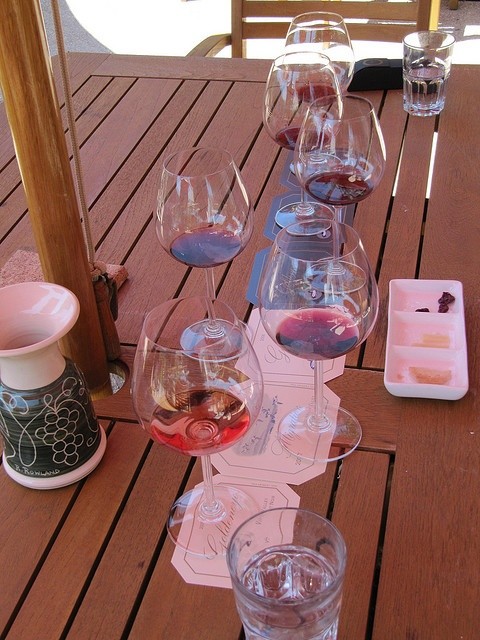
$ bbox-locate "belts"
[155,146,251,362]
[258,219,380,462]
[262,52,344,236]
[284,11,354,178]
[131,296,262,555]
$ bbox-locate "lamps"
[232,0,440,60]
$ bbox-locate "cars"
[225,507,347,640]
[402,30,456,116]
[294,95,387,294]
